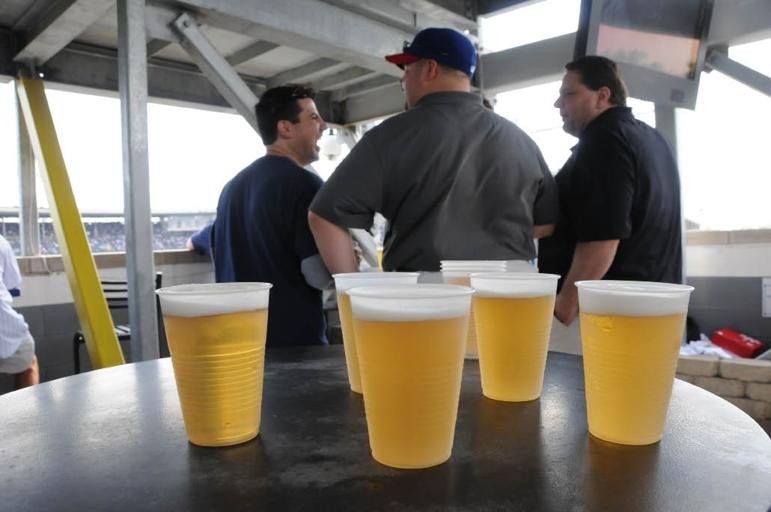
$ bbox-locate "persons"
[536,53,684,328]
[211,85,364,346]
[0,232,41,390]
[185,221,213,255]
[305,27,561,276]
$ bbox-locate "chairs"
[74,270,161,374]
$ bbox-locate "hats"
[385,27,478,78]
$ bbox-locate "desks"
[0,344,769,506]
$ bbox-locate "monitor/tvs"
[574,0,716,113]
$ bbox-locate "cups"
[157,281,272,448]
[571,277,695,448]
[331,258,565,469]
